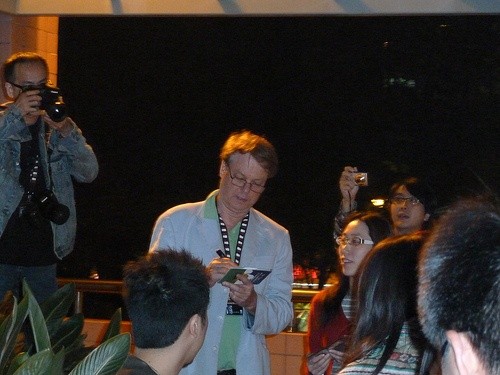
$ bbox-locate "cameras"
[352,172,369,186]
[27,191,70,230]
[24,84,70,122]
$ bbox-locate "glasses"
[387,195,420,207]
[336,236,374,247]
[228,165,267,195]
[8,80,53,92]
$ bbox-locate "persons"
[0,52,100,303]
[416,190,500,375]
[149,131,293,375]
[333,167,433,245]
[336,230,429,375]
[115,246,210,375]
[300,210,390,375]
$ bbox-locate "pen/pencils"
[215,250,228,256]
